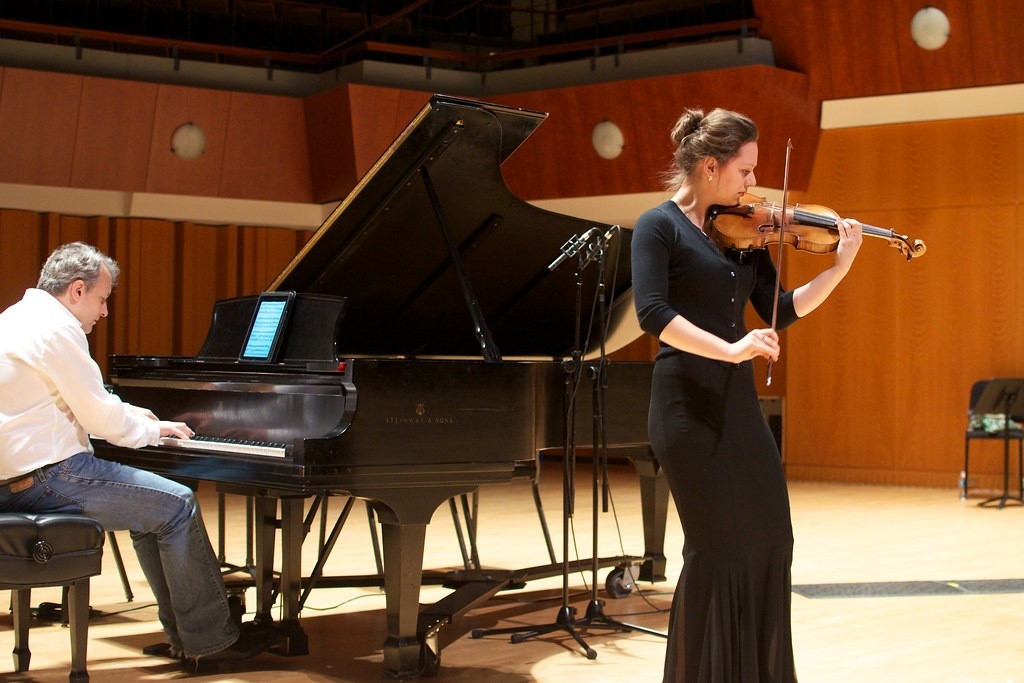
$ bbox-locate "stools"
[0,512,105,683]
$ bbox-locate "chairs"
[964,380,1024,500]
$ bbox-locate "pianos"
[88,93,671,683]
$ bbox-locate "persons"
[630,108,862,683]
[0,243,271,673]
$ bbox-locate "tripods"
[472,245,671,661]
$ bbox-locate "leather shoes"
[168,595,246,659]
[185,621,271,674]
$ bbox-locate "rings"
[761,335,765,340]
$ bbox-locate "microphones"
[578,227,617,271]
[548,228,594,272]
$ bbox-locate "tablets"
[238,291,294,364]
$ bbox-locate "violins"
[702,191,928,263]
